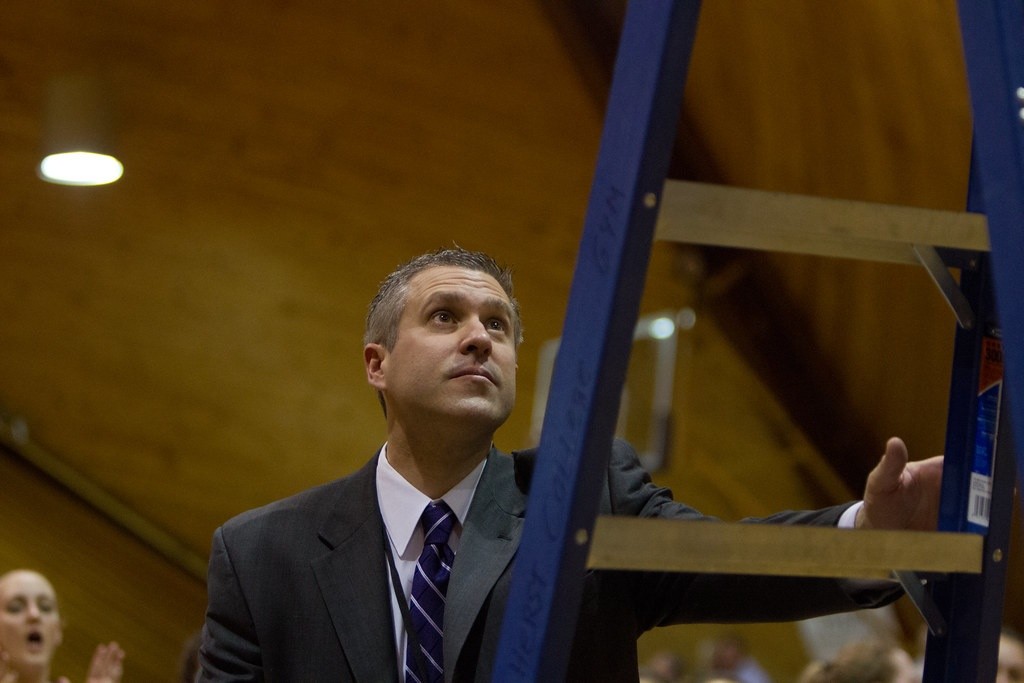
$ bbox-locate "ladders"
[493,0,1024,683]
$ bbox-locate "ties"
[405,501,458,683]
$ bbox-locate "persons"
[196,244,948,683]
[0,566,128,682]
